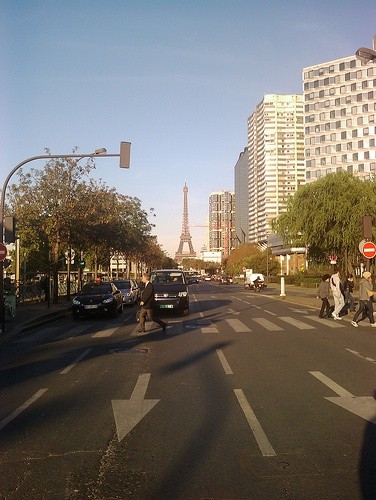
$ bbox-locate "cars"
[109,280,138,305]
[73,281,124,319]
[188,277,199,285]
[220,276,230,285]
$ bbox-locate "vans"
[244,274,264,289]
[148,269,189,317]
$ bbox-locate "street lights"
[66,148,106,302]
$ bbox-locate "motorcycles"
[253,280,263,291]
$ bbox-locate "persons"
[254,277,261,290]
[136,273,167,333]
[40,276,52,302]
[351,270,376,328]
[342,274,356,313]
[316,273,334,320]
[330,268,346,321]
[357,272,376,317]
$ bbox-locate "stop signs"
[0,243,7,261]
[362,242,376,258]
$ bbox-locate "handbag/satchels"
[367,289,376,298]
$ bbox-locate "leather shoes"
[162,323,166,332]
[135,328,146,333]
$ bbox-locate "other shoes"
[318,315,326,318]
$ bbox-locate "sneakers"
[332,313,342,321]
[350,320,358,328]
[370,321,376,328]
